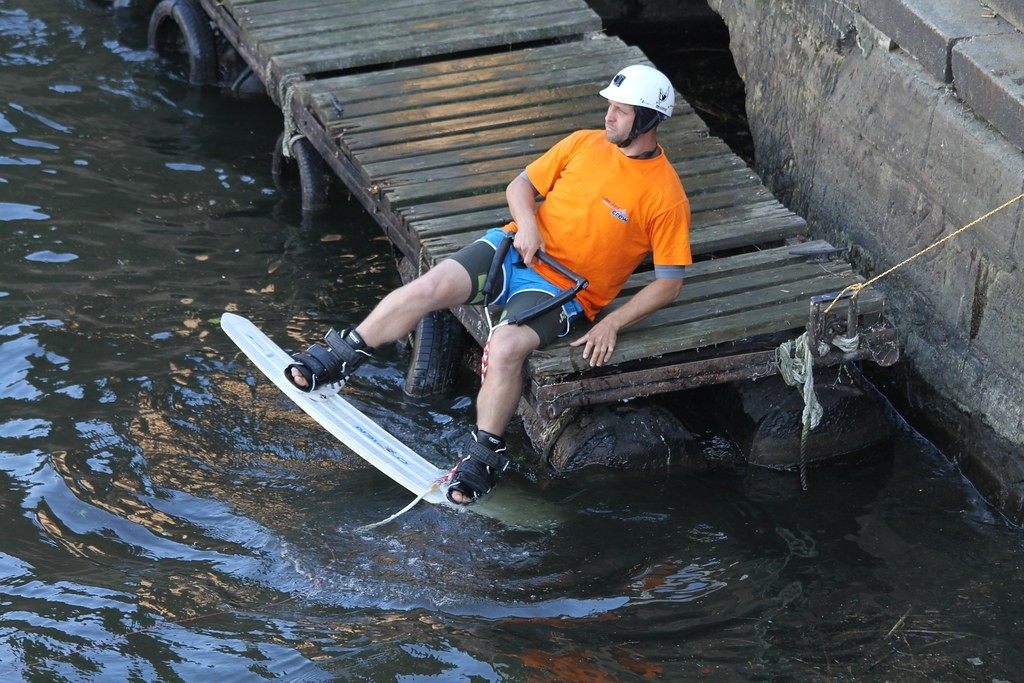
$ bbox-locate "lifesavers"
[146,0,219,83]
[273,131,331,210]
[394,309,453,398]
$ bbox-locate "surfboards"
[220,310,440,504]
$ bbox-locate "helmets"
[599,65,675,118]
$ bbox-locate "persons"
[284,63,693,506]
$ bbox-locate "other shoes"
[284,327,375,393]
[446,428,510,505]
